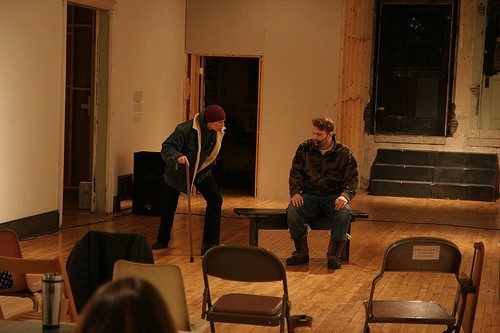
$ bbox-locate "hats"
[205,105,225,122]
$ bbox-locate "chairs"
[0,230,46,311]
[363,235,485,333]
[202,245,312,333]
[0,257,82,324]
[112,260,191,333]
[62,231,154,314]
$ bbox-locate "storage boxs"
[78,182,92,210]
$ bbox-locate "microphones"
[316,131,329,144]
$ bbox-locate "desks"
[0,320,77,333]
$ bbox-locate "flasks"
[41,273,64,330]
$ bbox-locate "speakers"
[132,151,166,216]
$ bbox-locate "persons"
[151,104,227,256]
[71,275,177,333]
[285,115,358,269]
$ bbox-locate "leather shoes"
[286,255,309,265]
[327,256,341,269]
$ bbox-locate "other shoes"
[151,240,168,249]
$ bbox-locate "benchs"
[233,204,369,261]
